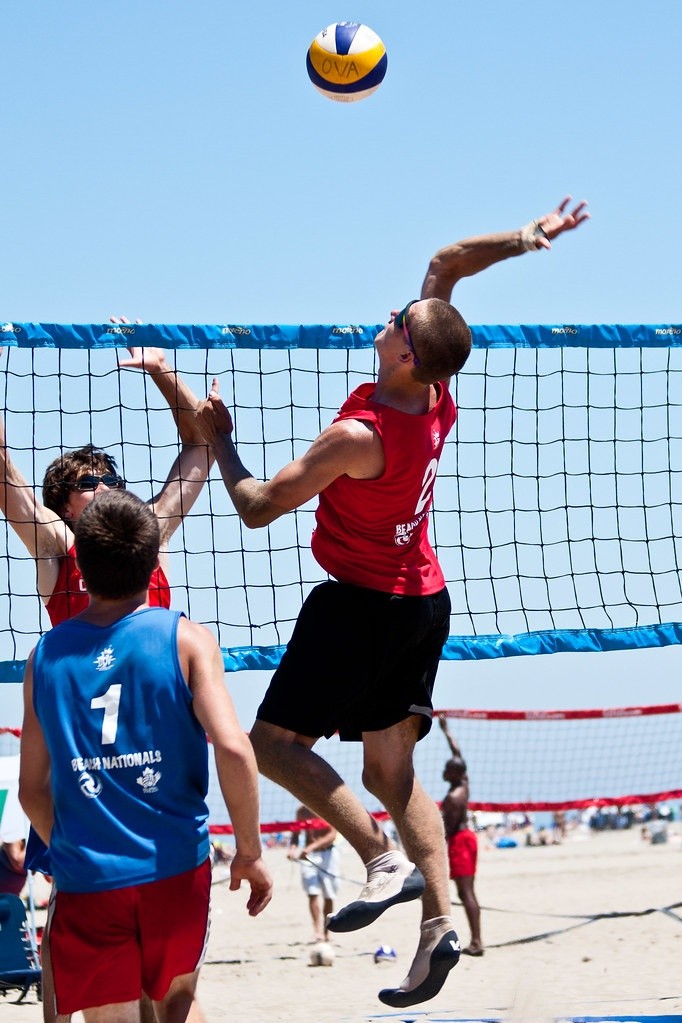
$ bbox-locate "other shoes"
[460,945,484,956]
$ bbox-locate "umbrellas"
[0,756,42,965]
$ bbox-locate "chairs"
[0,893,44,1005]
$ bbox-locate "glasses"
[63,472,125,505]
[395,300,424,369]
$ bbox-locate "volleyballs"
[304,21,389,105]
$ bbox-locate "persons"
[287,807,341,943]
[468,800,682,848]
[0,315,216,629]
[17,492,271,1023]
[439,713,485,956]
[0,841,55,970]
[194,193,589,1008]
